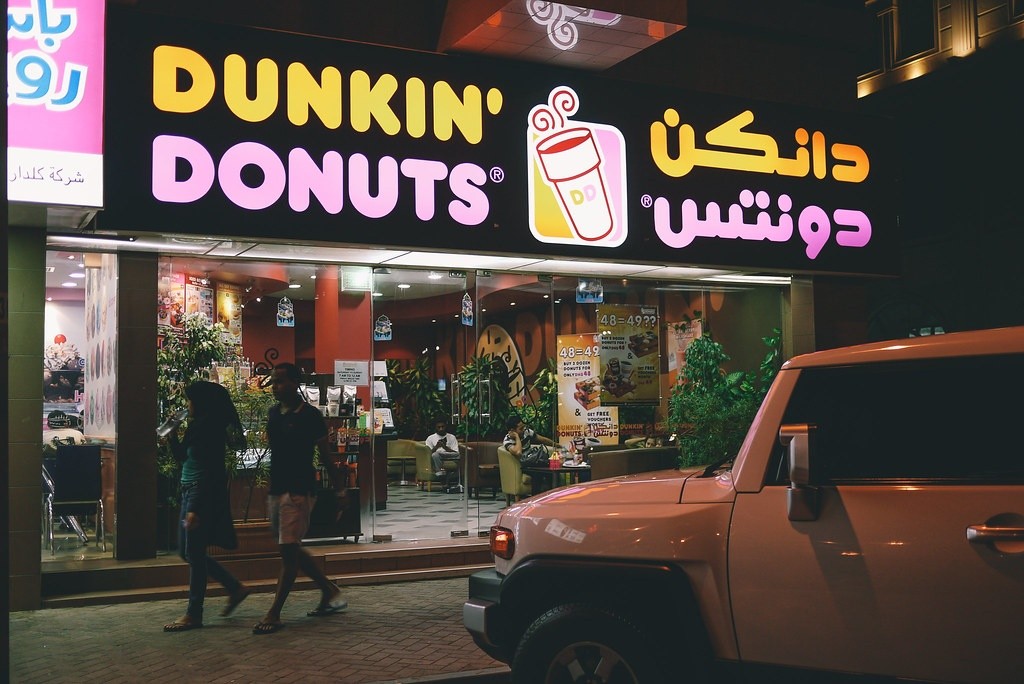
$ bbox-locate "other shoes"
[440,476,446,485]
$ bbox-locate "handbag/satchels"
[43,429,86,450]
[520,444,549,467]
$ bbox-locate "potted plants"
[207,375,283,560]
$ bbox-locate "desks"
[388,456,416,486]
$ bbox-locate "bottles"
[225,356,250,380]
[328,427,336,451]
[156,409,189,436]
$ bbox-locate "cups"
[610,360,620,375]
[574,437,585,446]
[585,437,600,446]
[620,361,632,378]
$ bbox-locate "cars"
[461,324,1024,684]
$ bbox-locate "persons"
[624,436,667,449]
[161,381,250,632]
[502,416,565,495]
[426,421,461,494]
[251,363,348,635]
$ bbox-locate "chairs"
[459,441,502,499]
[498,445,570,509]
[387,440,417,479]
[524,467,591,491]
[42,444,107,556]
[415,440,463,492]
[588,446,679,480]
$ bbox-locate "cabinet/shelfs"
[303,416,362,543]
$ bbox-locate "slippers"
[218,589,252,616]
[253,621,284,633]
[164,617,203,631]
[307,601,347,616]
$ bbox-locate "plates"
[563,460,587,467]
